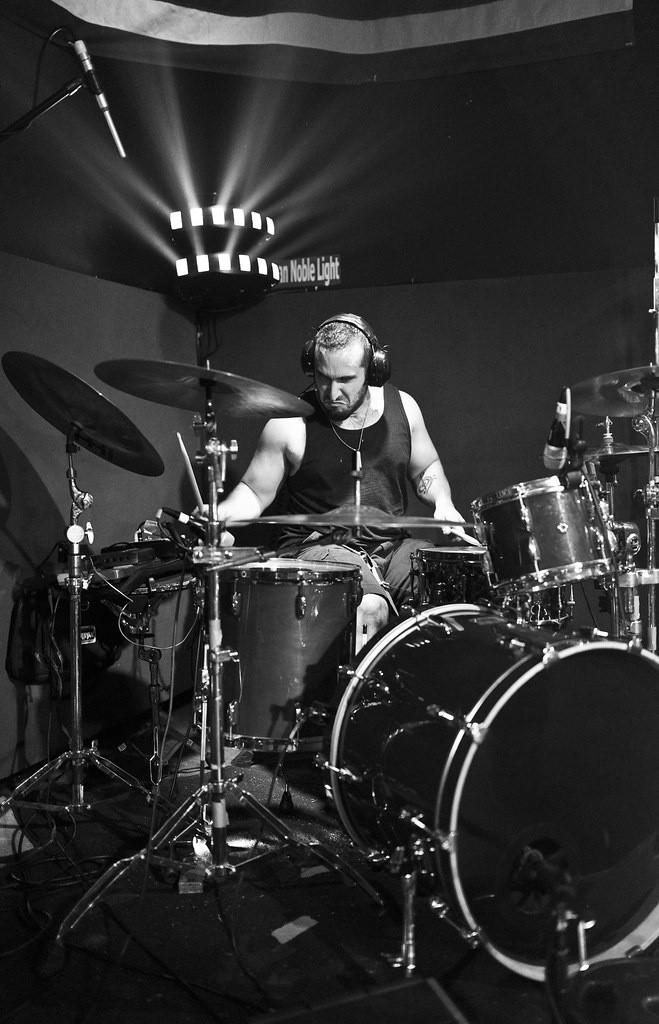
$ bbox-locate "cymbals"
[562,364,659,419]
[577,443,659,456]
[94,358,315,419]
[232,505,490,529]
[2,350,166,476]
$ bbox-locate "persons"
[192,314,467,807]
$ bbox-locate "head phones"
[301,315,392,387]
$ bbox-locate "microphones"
[544,388,570,470]
[519,847,566,897]
[162,507,235,548]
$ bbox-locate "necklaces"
[331,406,369,472]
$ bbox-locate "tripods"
[0,382,402,984]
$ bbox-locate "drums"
[472,474,621,595]
[217,555,365,754]
[330,604,659,981]
[413,545,487,605]
